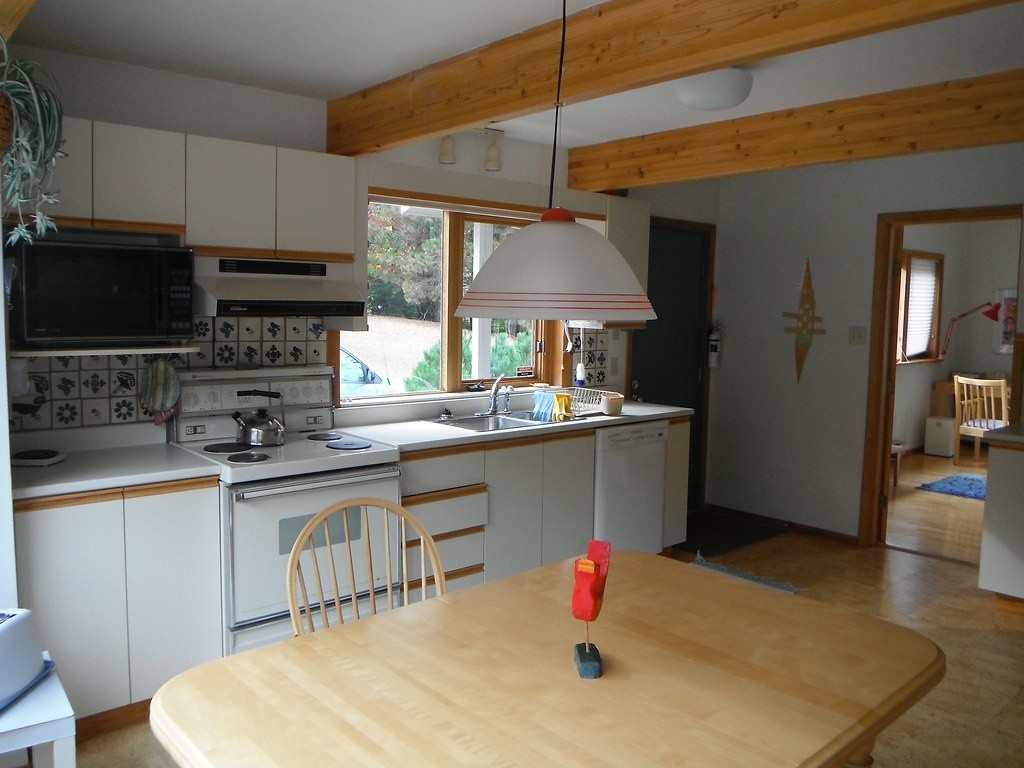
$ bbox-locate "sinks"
[504,410,581,423]
[439,417,537,430]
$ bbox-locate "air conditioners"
[454,1,659,320]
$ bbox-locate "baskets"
[545,387,624,417]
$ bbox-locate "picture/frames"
[991,288,1020,355]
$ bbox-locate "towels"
[532,390,553,420]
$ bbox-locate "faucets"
[488,374,504,414]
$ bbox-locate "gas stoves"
[169,365,400,482]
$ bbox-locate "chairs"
[286,497,447,637]
[952,376,1005,464]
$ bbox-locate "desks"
[150,547,947,767]
[932,379,1012,418]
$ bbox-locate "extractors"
[193,256,364,319]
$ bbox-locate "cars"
[340,339,394,398]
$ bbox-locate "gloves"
[552,394,575,423]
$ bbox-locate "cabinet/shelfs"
[401,443,488,603]
[14,477,224,736]
[0,115,356,266]
[483,433,600,587]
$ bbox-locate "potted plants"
[0,35,69,245]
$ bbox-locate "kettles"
[232,390,285,445]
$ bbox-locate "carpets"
[668,512,783,561]
[914,471,990,499]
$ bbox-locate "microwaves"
[2,223,194,352]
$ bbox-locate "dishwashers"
[593,419,668,554]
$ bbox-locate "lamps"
[939,300,1002,360]
[440,135,456,164]
[485,134,503,172]
[674,66,754,111]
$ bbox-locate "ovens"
[218,465,403,657]
